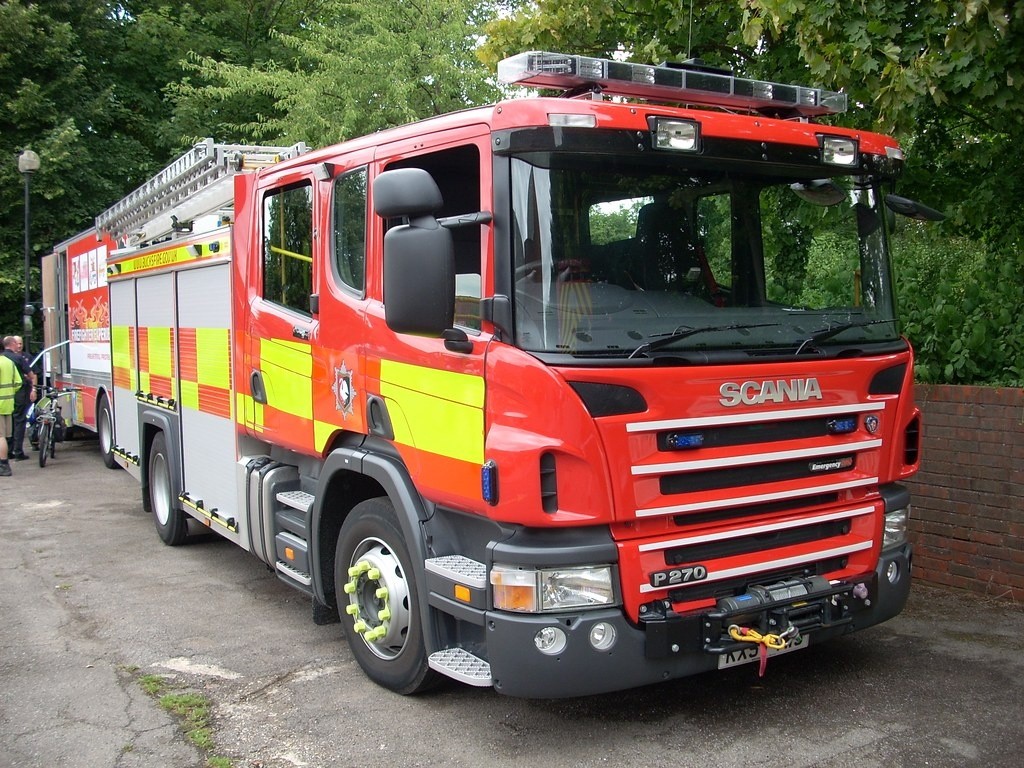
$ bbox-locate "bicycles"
[31,382,83,468]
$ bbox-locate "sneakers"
[0,463,13,476]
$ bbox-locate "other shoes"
[32,443,40,451]
[7,452,16,459]
[16,452,29,460]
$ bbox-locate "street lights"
[17,149,42,411]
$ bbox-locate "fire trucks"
[34,48,952,700]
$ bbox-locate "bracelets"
[32,388,38,392]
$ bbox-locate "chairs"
[622,203,700,293]
[431,178,480,273]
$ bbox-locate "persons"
[0,336,39,475]
